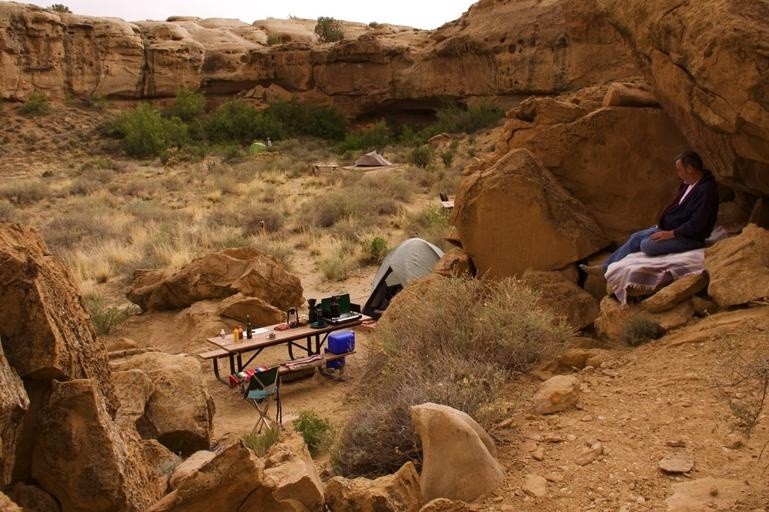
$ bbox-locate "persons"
[578,150,719,280]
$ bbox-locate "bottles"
[219,328,226,345]
[246,317,252,339]
[233,324,244,343]
[307,298,317,323]
[287,306,299,328]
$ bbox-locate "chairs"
[239,364,282,436]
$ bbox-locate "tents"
[360,238,445,328]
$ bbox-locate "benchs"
[196,350,355,404]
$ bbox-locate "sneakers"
[580,264,606,283]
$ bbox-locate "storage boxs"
[324,331,355,369]
